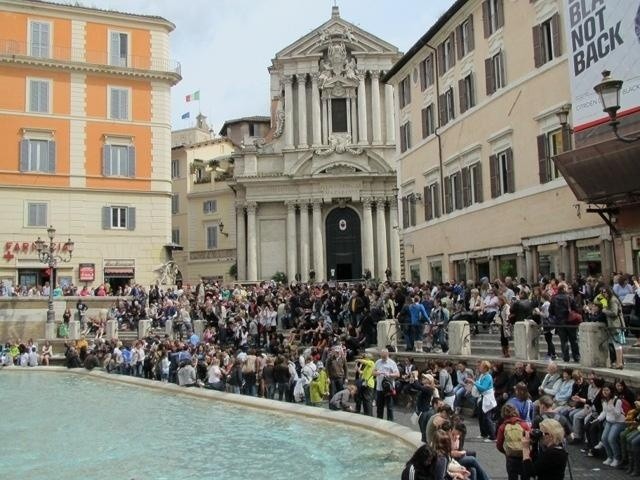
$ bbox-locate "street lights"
[33,221,77,340]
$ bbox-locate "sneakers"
[580,441,603,457]
[484,439,496,442]
[570,432,581,439]
[602,457,639,476]
[477,435,484,439]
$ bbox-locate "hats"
[317,361,323,368]
[334,346,340,353]
[312,372,319,378]
[422,374,433,383]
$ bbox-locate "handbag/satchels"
[381,376,396,396]
[471,385,480,398]
[568,311,583,324]
[410,412,419,425]
[418,311,426,322]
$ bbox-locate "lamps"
[555,106,574,134]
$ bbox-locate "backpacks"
[503,421,524,457]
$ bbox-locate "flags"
[185,90,199,102]
[181,111,189,119]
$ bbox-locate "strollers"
[447,309,479,336]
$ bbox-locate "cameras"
[523,428,543,440]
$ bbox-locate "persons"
[0,271,640,480]
